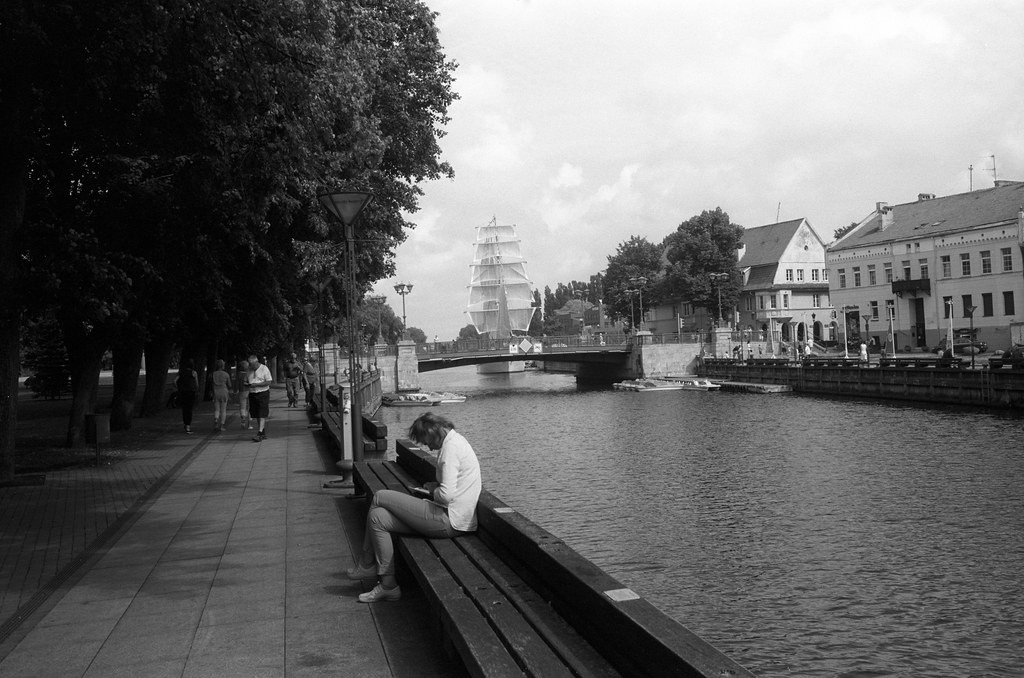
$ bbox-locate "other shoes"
[248,426,253,429]
[219,427,226,430]
[252,435,263,441]
[294,402,297,407]
[184,426,191,433]
[288,401,292,407]
[263,434,268,438]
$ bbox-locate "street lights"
[371,292,388,344]
[575,288,591,335]
[302,303,317,360]
[394,281,413,341]
[709,271,731,328]
[737,322,746,365]
[316,189,376,500]
[322,310,346,385]
[966,305,978,370]
[623,289,639,333]
[862,314,872,368]
[630,276,651,331]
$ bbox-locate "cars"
[931,335,988,356]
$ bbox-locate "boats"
[612,374,721,393]
[382,387,467,408]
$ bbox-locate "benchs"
[705,357,740,365]
[746,358,795,366]
[320,411,376,463]
[802,358,865,368]
[352,460,622,678]
[988,357,1024,369]
[879,357,962,368]
[309,394,336,426]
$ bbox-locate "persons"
[747,327,752,335]
[758,327,763,340]
[804,343,810,356]
[302,356,319,405]
[723,340,762,359]
[938,348,951,358]
[691,327,704,342]
[232,361,254,430]
[860,341,867,362]
[1002,346,1023,358]
[242,355,273,441]
[346,412,482,603]
[208,359,232,432]
[173,358,199,434]
[543,333,547,345]
[284,353,303,408]
[434,335,439,341]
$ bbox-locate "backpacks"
[180,370,195,394]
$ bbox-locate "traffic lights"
[680,318,683,328]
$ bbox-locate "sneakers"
[346,565,377,579]
[359,584,401,603]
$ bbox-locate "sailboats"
[462,214,538,375]
